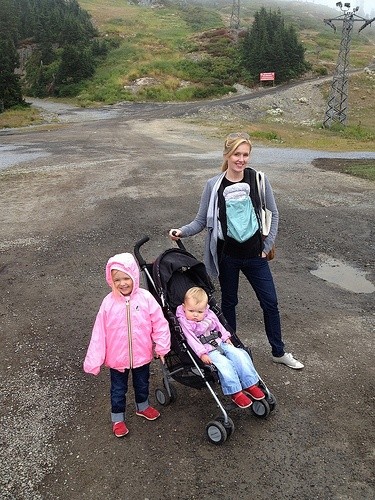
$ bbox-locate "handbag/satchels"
[256,171,272,236]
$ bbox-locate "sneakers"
[273,353,304,368]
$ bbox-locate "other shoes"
[245,386,265,400]
[136,406,160,421]
[113,421,129,437]
[232,393,252,407]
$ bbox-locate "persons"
[175,287,266,409]
[83,253,171,437]
[168,136,304,369]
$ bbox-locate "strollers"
[133,231,278,446]
